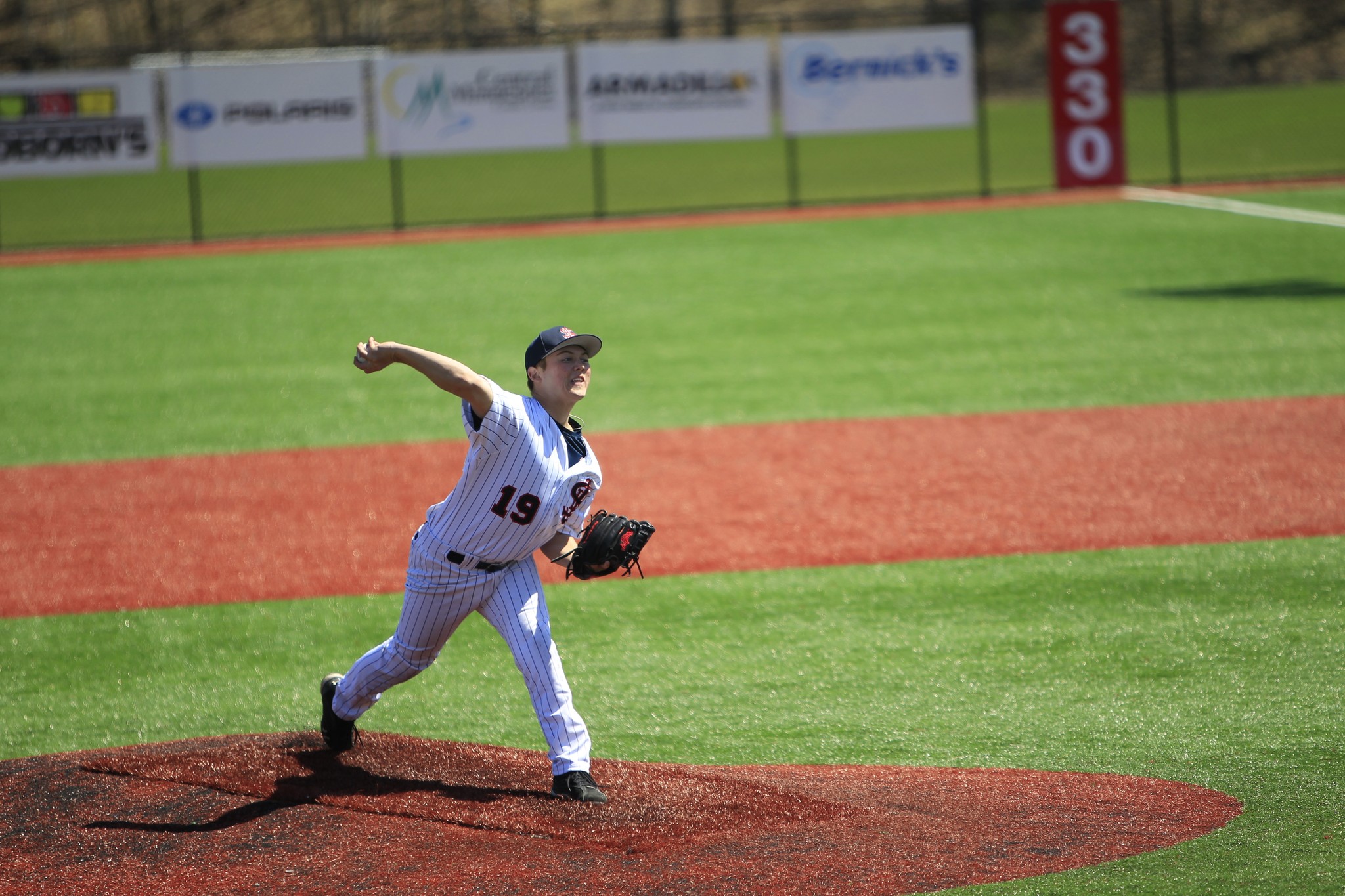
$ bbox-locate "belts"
[414,522,513,573]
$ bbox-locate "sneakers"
[551,770,608,804]
[321,673,365,752]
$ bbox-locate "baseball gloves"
[570,510,655,582]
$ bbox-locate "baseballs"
[356,342,377,362]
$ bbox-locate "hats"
[525,326,602,373]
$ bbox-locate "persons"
[321,325,657,805]
